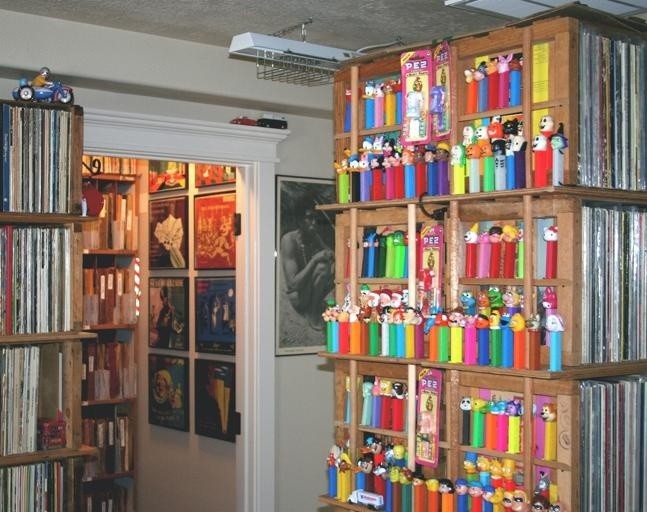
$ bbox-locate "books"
[82,154,138,512]
[0,102,72,512]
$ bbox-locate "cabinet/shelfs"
[83,152,138,512]
[1,98,84,512]
[319,0,647,512]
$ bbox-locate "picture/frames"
[147,159,237,442]
[275,174,336,357]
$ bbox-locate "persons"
[280,196,335,331]
[155,285,176,349]
